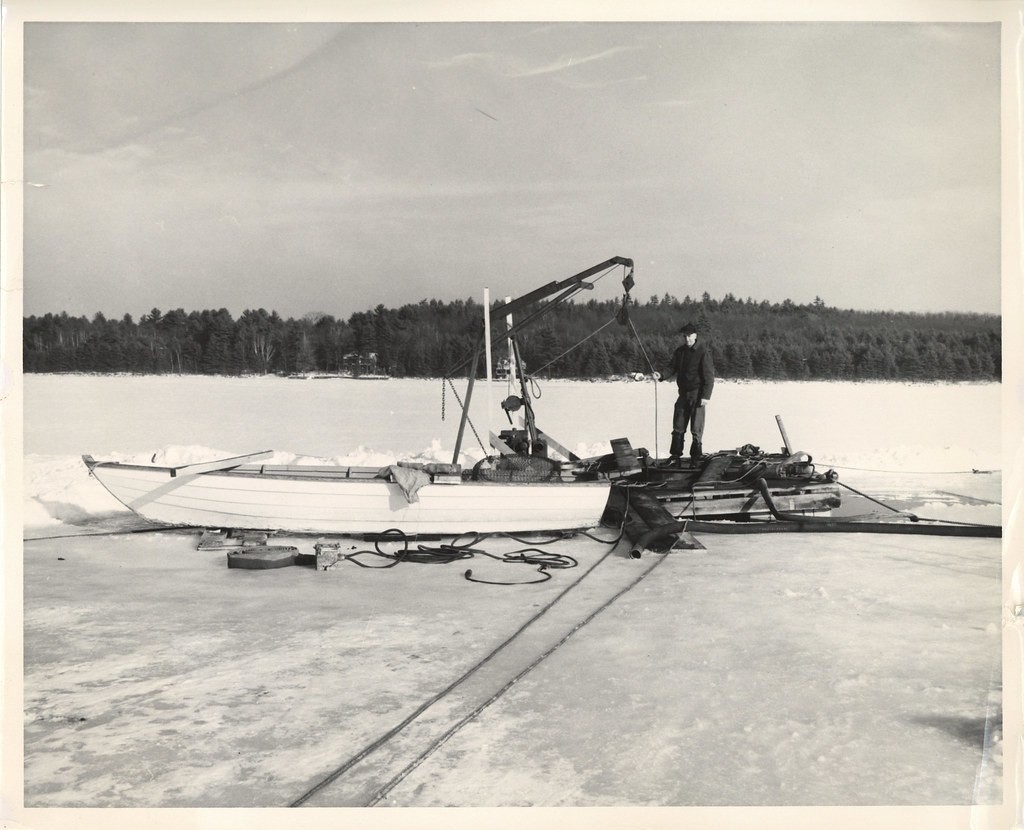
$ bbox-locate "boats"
[78,281,842,541]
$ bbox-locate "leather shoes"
[660,456,681,468]
[689,459,699,469]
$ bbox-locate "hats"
[680,324,697,334]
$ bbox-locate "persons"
[652,322,717,470]
